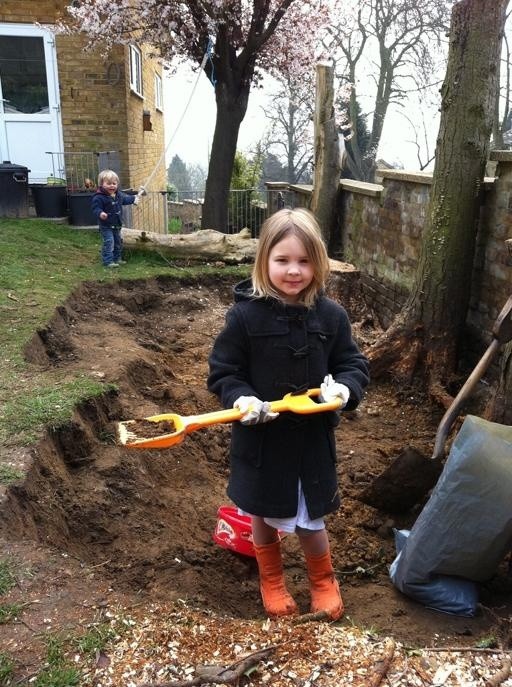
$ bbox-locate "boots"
[305,544,344,620]
[252,535,298,618]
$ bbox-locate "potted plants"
[67,177,98,226]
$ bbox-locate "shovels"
[118,387,343,447]
[353,294,512,514]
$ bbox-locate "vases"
[28,183,67,217]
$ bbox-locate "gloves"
[319,374,350,410]
[233,396,279,426]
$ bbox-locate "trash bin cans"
[32,183,94,224]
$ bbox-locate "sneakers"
[107,260,127,267]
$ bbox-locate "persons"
[89,168,142,269]
[205,205,373,625]
